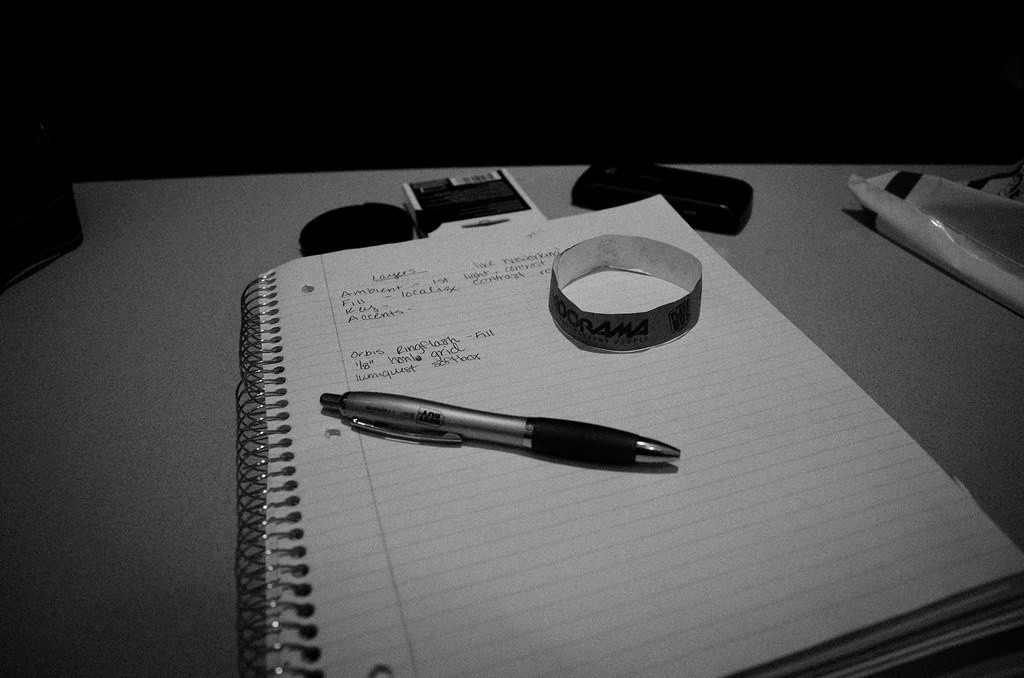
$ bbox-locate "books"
[234,192,1024,678]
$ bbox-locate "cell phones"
[572,159,753,236]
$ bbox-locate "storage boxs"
[402,167,548,240]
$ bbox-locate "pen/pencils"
[319,391,681,470]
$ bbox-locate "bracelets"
[550,234,703,350]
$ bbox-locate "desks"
[0,161,1024,678]
